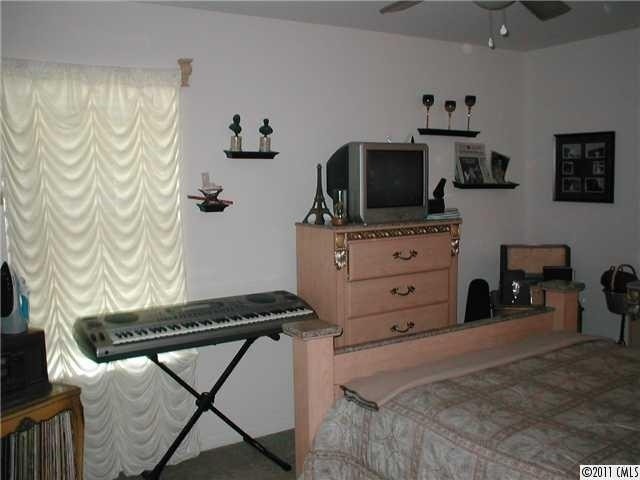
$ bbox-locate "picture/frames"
[554,129,615,204]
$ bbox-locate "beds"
[282,278,639,480]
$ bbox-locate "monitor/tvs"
[326,142,430,223]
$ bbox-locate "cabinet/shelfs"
[296,216,463,349]
[418,127,520,189]
[196,149,280,211]
[0,383,86,480]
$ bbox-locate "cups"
[332,190,348,227]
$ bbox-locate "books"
[426,207,463,221]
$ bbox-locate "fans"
[378,1,573,21]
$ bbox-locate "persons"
[492,157,505,183]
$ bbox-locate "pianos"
[73,289,319,363]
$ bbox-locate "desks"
[492,289,584,333]
